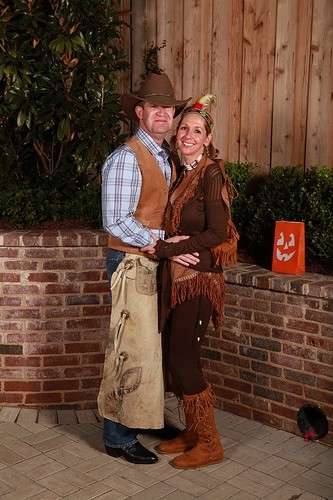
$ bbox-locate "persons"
[140,107,230,468]
[101,72,200,465]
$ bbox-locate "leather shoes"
[104,441,159,464]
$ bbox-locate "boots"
[169,382,224,469]
[156,412,197,454]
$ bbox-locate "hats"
[120,73,191,122]
[181,94,217,132]
